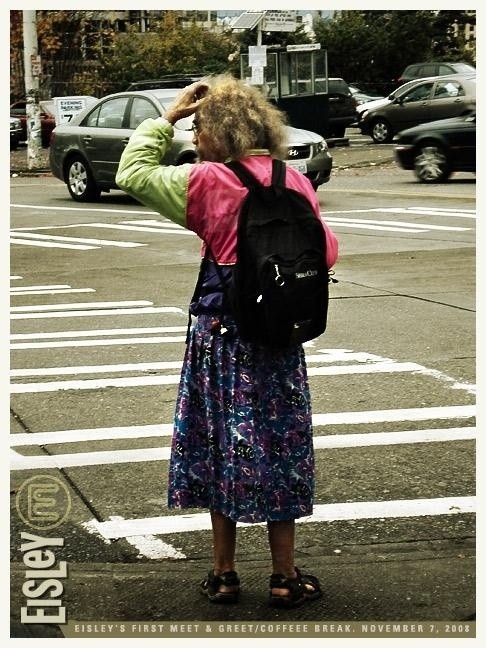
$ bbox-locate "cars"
[10,101,55,150]
[268,62,476,183]
[49,88,332,202]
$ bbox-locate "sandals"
[199,570,240,601]
[272,568,319,596]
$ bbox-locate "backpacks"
[234,157,329,348]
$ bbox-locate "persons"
[112,75,340,607]
